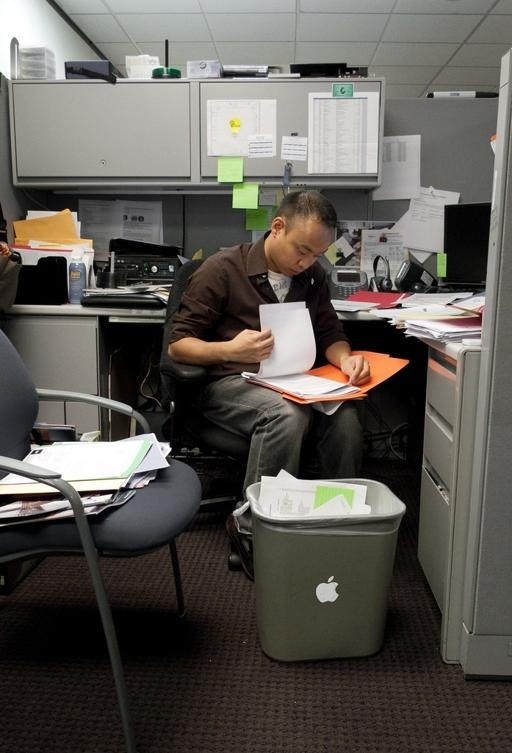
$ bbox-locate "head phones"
[369,254,394,291]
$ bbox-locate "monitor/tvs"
[438,201,492,291]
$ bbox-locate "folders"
[243,350,410,404]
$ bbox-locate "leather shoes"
[224,510,254,583]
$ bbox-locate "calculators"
[330,268,368,301]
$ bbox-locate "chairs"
[161,259,250,571]
[0,324,206,747]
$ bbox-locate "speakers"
[394,259,437,295]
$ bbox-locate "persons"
[158,189,371,581]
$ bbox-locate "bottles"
[69,251,86,304]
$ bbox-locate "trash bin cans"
[245,478,408,662]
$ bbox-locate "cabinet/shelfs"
[7,77,382,192]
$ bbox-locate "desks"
[5,290,482,689]
